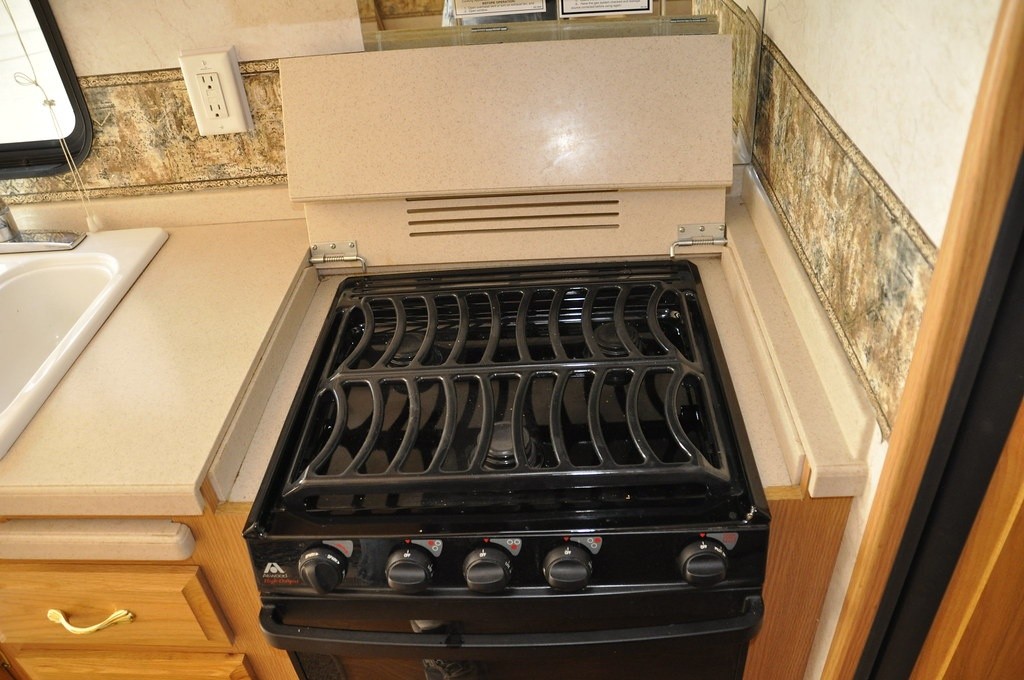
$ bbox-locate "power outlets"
[177,45,255,139]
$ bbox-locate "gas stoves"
[242,261,771,614]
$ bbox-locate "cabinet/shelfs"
[0,503,291,680]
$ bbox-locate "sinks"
[0,227,169,462]
